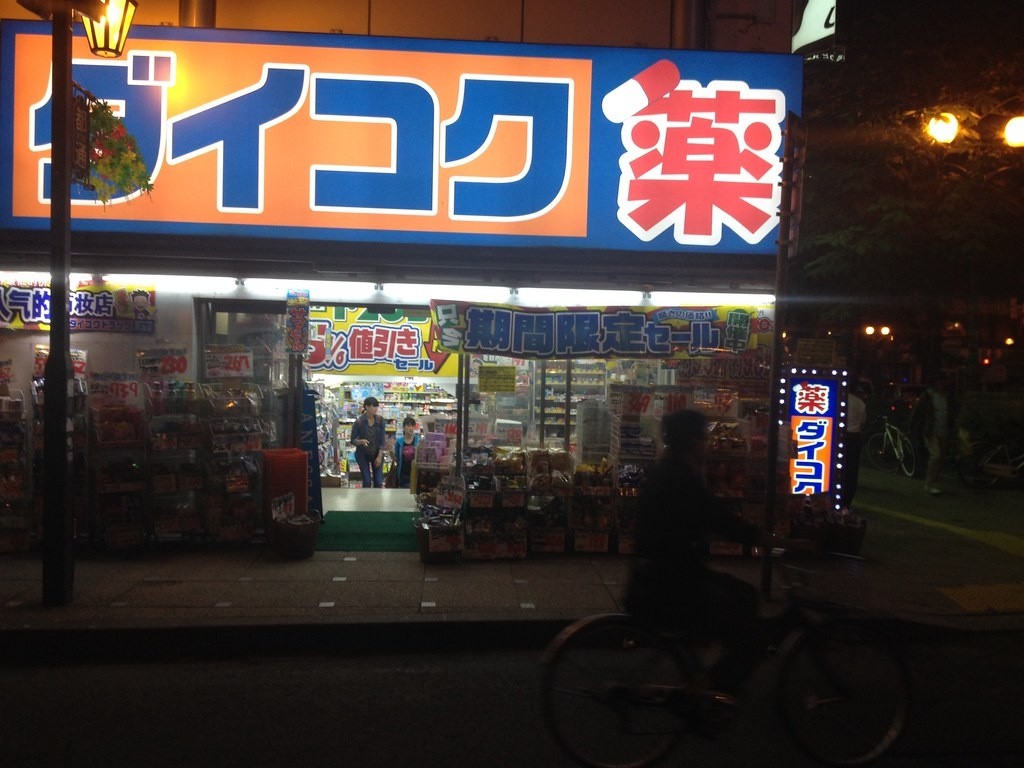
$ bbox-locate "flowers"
[89,102,155,215]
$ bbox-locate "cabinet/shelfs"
[339,390,439,481]
[0,409,36,553]
[532,358,607,427]
[86,404,149,553]
[144,414,206,553]
[202,416,264,548]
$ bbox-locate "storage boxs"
[575,385,738,467]
[262,448,309,548]
[416,417,634,561]
[0,422,255,552]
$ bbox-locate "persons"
[388,417,420,488]
[627,410,821,768]
[840,375,866,508]
[350,397,386,488]
[908,373,954,494]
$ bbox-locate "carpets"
[315,510,421,552]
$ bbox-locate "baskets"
[276,509,322,560]
[788,517,868,565]
[413,514,463,564]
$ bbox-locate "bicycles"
[866,411,917,477]
[956,423,1024,491]
[530,534,917,768]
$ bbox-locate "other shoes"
[926,485,940,494]
